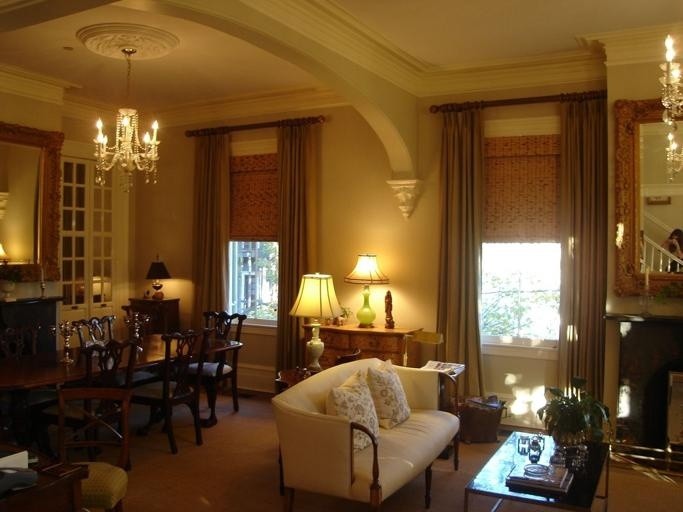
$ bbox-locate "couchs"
[272,357,461,512]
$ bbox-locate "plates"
[523,463,550,476]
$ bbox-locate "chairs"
[58,387,133,512]
[0,304,247,461]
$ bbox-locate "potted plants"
[537,377,612,467]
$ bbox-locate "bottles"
[518,431,546,461]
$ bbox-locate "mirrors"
[612,98,683,299]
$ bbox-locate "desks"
[302,320,423,369]
[0,441,89,512]
[128,297,180,334]
[275,366,332,496]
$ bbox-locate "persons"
[662,227,683,272]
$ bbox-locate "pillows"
[327,359,411,454]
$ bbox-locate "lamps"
[145,252,171,300]
[658,34,683,183]
[660,114,682,179]
[92,49,161,193]
[289,272,344,369]
[344,254,390,328]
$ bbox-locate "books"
[31,454,63,470]
[505,470,575,495]
[44,462,83,477]
[506,461,568,487]
